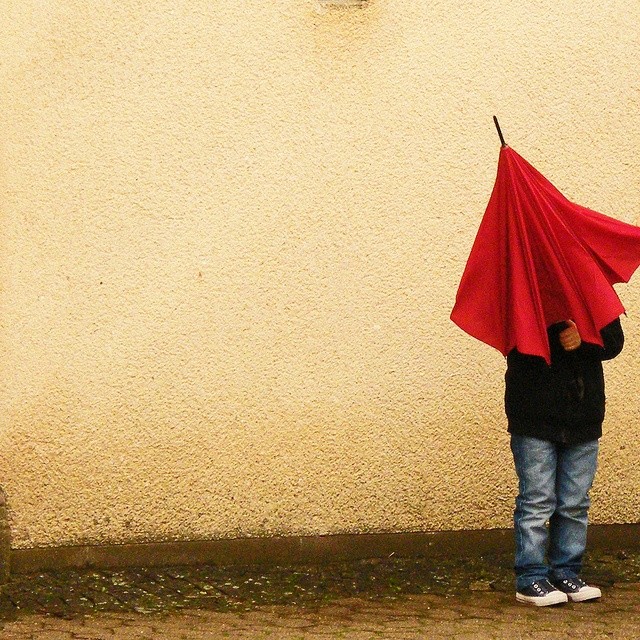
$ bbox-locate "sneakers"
[515,579,568,606]
[550,576,602,602]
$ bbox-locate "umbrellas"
[448,115,640,367]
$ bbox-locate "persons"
[505,317,624,607]
